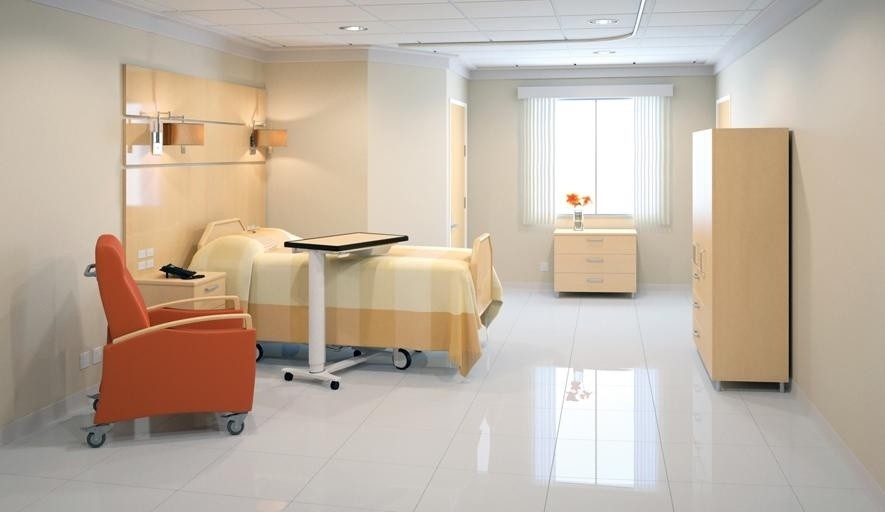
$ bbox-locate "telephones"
[160,263,196,279]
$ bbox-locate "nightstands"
[133,271,227,310]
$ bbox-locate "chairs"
[82,234,257,447]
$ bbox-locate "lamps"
[250,128,288,155]
[151,111,206,155]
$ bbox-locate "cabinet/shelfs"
[552,213,638,298]
[690,126,791,394]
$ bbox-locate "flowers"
[565,193,593,208]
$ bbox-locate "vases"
[573,209,584,231]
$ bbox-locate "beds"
[186,218,504,378]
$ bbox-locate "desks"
[281,231,411,390]
